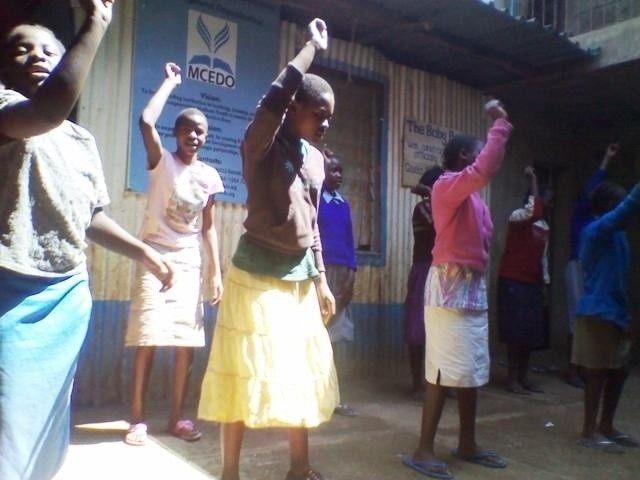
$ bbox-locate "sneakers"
[284,468,323,480]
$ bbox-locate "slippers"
[503,383,530,396]
[451,447,508,468]
[523,382,543,393]
[167,420,202,441]
[575,435,625,453]
[604,431,634,446]
[401,454,453,478]
[124,423,147,445]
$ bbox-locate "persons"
[565,143,625,389]
[494,163,552,396]
[404,167,460,403]
[196,18,341,480]
[570,178,639,452]
[402,97,510,478]
[0,0,176,479]
[123,59,223,444]
[319,145,359,417]
